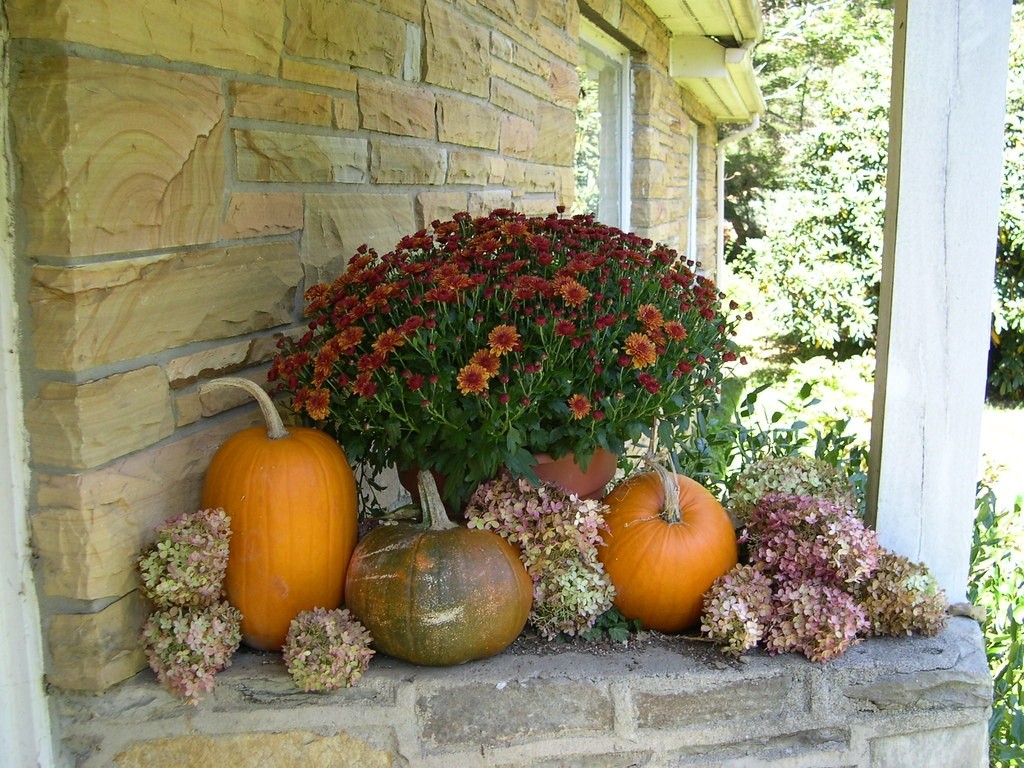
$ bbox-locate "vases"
[397,445,617,523]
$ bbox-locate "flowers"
[139,509,245,707]
[462,472,617,640]
[267,205,755,522]
[698,458,950,661]
[282,608,376,694]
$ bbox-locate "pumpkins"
[599,458,739,632]
[196,378,357,653]
[346,467,533,667]
